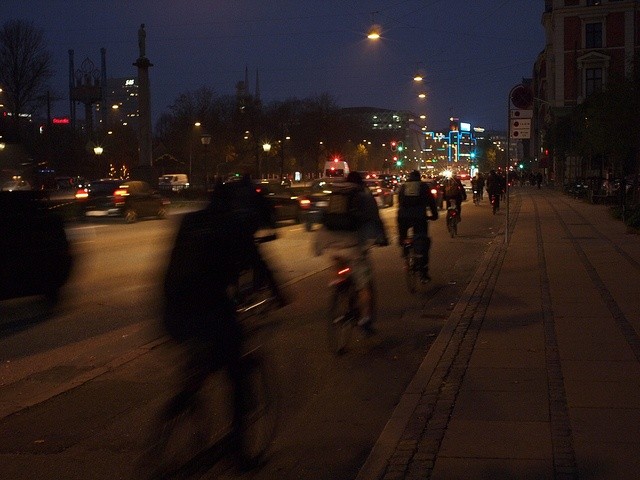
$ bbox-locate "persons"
[396,169,438,286]
[312,171,388,332]
[444,173,467,221]
[470,168,545,210]
[154,176,288,414]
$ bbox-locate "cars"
[2,181,32,191]
[362,178,393,209]
[253,179,306,230]
[0,186,73,307]
[356,160,472,185]
[74,178,172,225]
[296,177,346,231]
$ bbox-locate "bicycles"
[473,188,480,207]
[141,234,281,478]
[400,215,439,297]
[446,200,457,238]
[490,194,500,215]
[325,237,390,356]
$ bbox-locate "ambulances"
[322,158,349,180]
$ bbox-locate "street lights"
[200,131,213,210]
